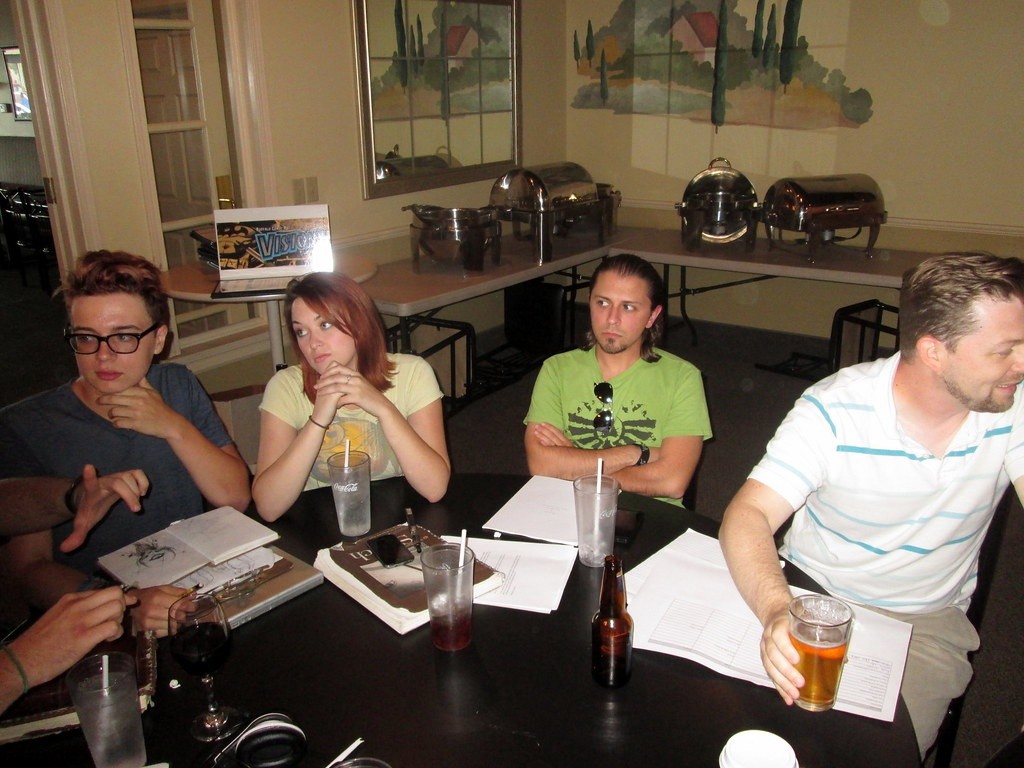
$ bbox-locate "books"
[0,633,158,746]
[315,521,505,634]
[96,506,281,589]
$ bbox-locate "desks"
[608,230,943,348]
[357,224,659,354]
[159,250,377,373]
[0,471,924,768]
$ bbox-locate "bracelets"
[309,416,329,429]
[633,443,649,464]
[1,644,28,692]
[78,576,137,593]
[64,475,83,514]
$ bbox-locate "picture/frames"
[0,46,32,122]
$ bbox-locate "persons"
[524,254,714,509]
[720,244,1024,760]
[0,249,250,639]
[253,272,452,521]
[0,463,150,711]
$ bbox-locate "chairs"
[0,187,57,299]
[753,298,900,383]
[475,271,592,379]
[385,315,518,420]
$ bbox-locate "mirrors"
[349,0,522,202]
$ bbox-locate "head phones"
[194,713,393,768]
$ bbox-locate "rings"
[346,375,352,383]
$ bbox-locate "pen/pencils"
[181,580,204,600]
[404,505,424,554]
[120,584,129,589]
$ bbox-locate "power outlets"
[305,176,320,204]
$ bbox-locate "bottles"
[592,556,632,689]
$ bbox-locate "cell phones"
[365,534,415,568]
[614,507,643,545]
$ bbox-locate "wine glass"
[168,594,242,742]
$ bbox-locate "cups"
[573,474,618,567]
[788,595,853,712]
[66,652,147,768]
[719,730,800,768]
[332,757,392,768]
[420,544,474,651]
[327,451,371,536]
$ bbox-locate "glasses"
[63,320,159,355]
